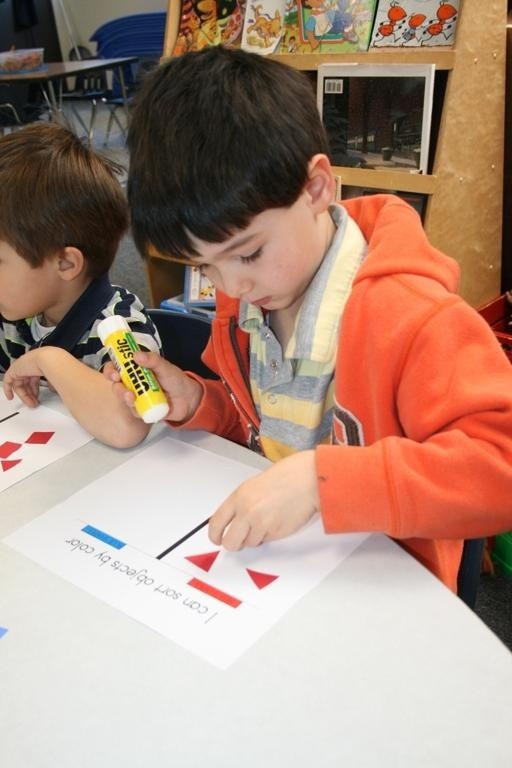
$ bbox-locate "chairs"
[0,45,158,147]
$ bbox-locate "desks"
[0,372,511,763]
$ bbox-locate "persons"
[100,44,511,550]
[0,124,166,450]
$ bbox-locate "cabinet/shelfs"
[141,0,510,313]
